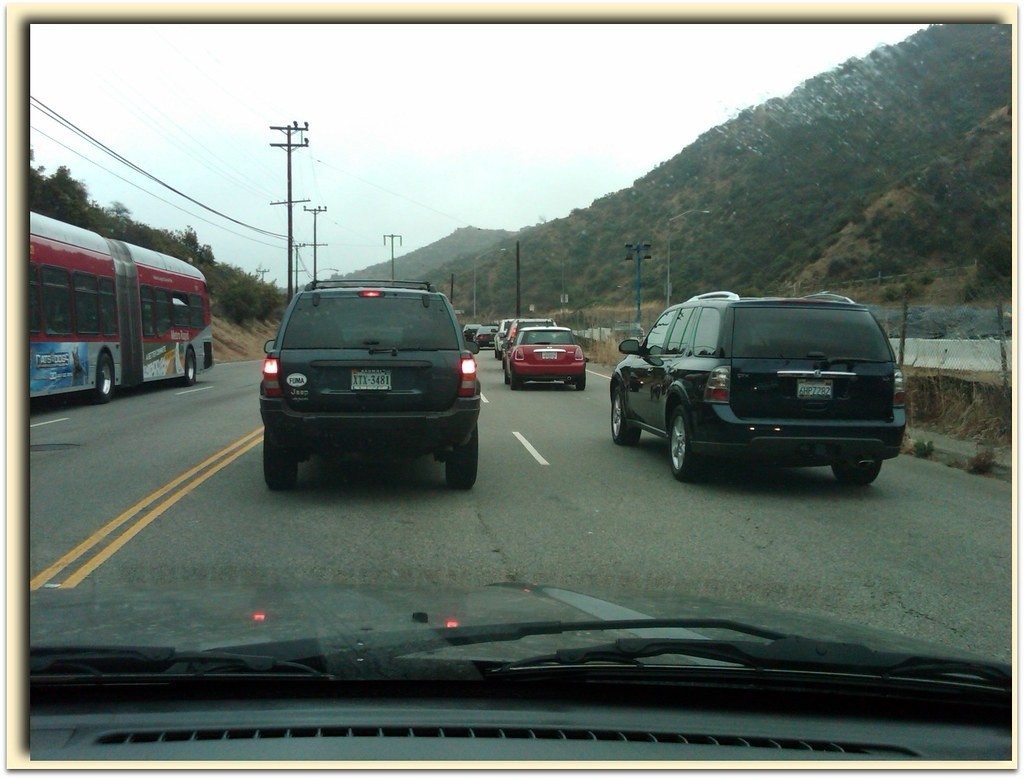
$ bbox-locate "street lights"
[622,238,653,342]
[473,246,507,324]
[665,209,713,312]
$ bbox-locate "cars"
[473,325,499,350]
[502,326,591,391]
[463,324,483,343]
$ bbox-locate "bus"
[29,210,215,403]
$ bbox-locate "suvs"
[491,318,559,371]
[258,278,480,492]
[610,287,911,486]
